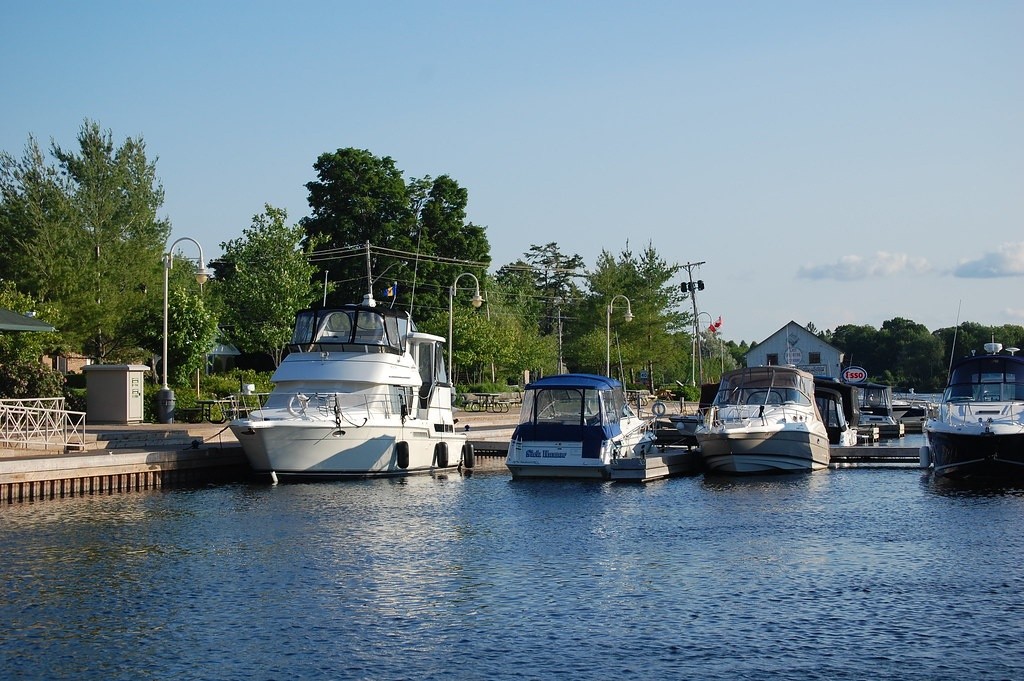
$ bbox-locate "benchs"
[463,401,480,412]
[493,401,509,413]
[182,407,209,423]
[221,408,252,424]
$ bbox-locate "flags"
[383,284,397,297]
[709,317,722,333]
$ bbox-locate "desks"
[197,400,239,424]
[475,394,502,412]
[626,390,649,406]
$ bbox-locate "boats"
[921,300,1024,486]
[228,223,476,487]
[695,320,831,479]
[809,373,938,446]
[503,304,698,483]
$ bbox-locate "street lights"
[155,237,212,424]
[607,295,636,378]
[449,273,486,387]
[693,311,713,388]
